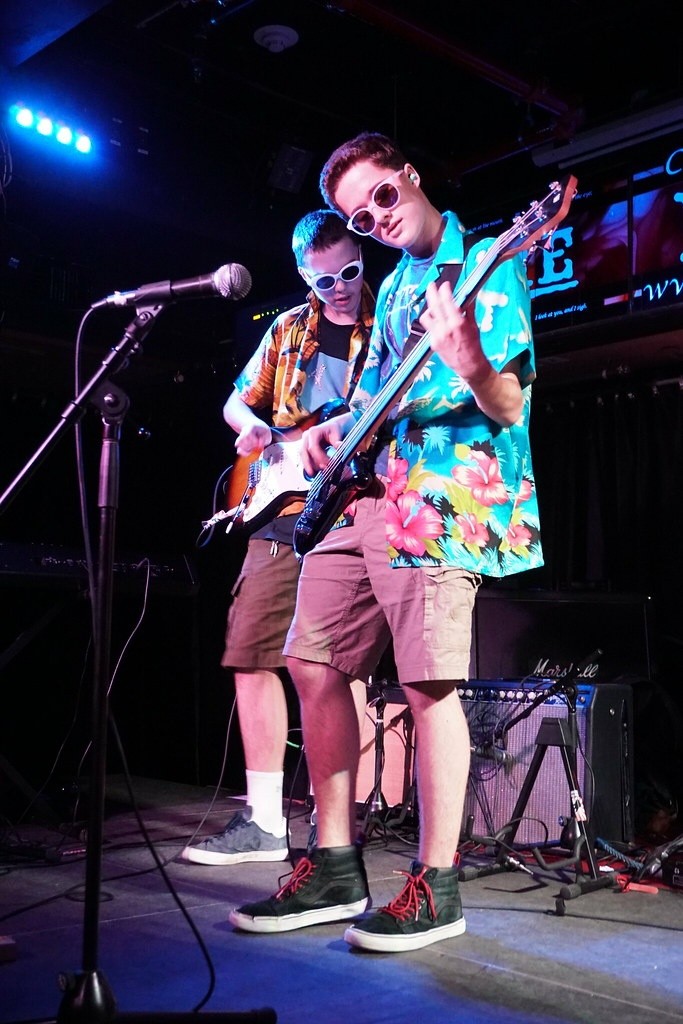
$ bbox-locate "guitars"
[290,172,581,557]
[198,400,347,549]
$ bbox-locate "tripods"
[0,300,280,1024]
[477,650,664,881]
[355,679,423,847]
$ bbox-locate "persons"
[183,209,367,863]
[230,136,546,952]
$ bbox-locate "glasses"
[302,244,364,292]
[346,169,404,237]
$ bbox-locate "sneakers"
[344,853,466,952]
[229,842,372,932]
[307,812,318,856]
[181,805,291,865]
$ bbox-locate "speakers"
[457,590,661,856]
[306,682,419,830]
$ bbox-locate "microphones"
[91,263,253,311]
[469,744,513,765]
